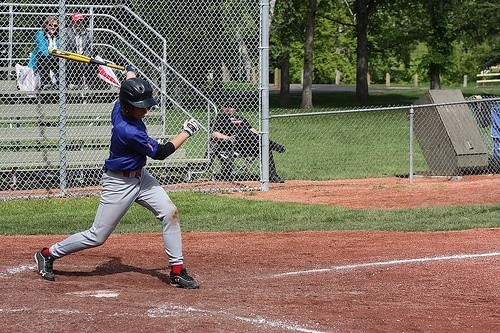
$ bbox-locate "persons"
[210,102,285,183]
[27,13,111,90]
[34,65,199,289]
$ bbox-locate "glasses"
[222,105,234,108]
[47,24,59,28]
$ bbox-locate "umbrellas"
[91,55,122,88]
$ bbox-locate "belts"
[123,170,141,178]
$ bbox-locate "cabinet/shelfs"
[407,89,489,176]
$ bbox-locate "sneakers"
[169,267,199,289]
[33,247,55,280]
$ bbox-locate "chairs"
[206,134,264,178]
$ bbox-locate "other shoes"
[269,141,287,153]
[268,175,283,184]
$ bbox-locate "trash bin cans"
[490,106,500,166]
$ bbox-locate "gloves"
[183,117,199,136]
[119,62,135,75]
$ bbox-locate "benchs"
[0,80,211,189]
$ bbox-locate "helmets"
[119,77,158,109]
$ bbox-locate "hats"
[70,12,84,21]
